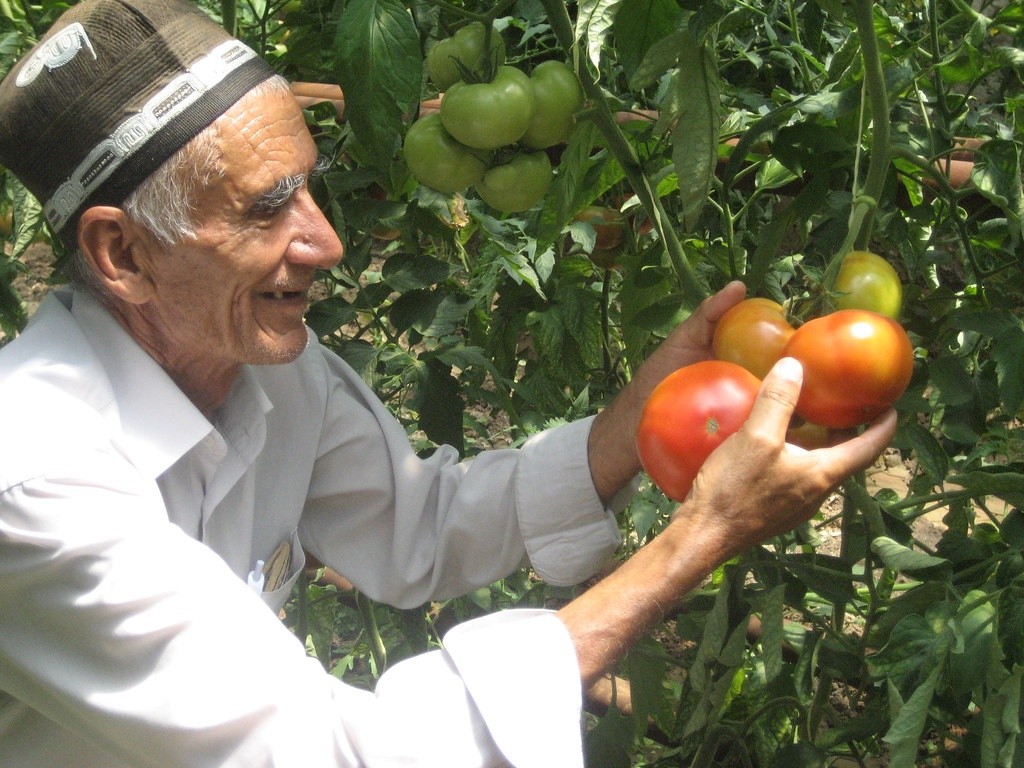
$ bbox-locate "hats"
[0,0,276,279]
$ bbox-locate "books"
[261,541,291,592]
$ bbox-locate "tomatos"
[638,249,914,502]
[400,20,625,251]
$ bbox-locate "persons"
[0,0,898,768]
